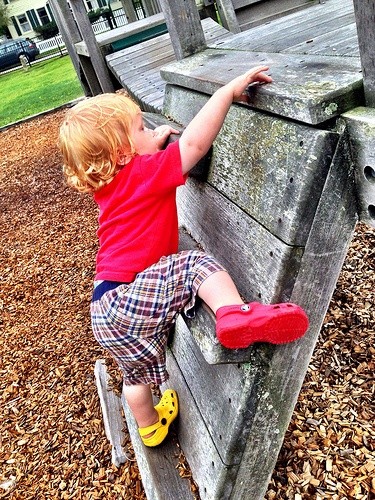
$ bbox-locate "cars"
[0,36,40,73]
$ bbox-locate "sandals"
[215,301,309,349]
[138,389,179,447]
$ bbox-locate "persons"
[56,66,308,448]
